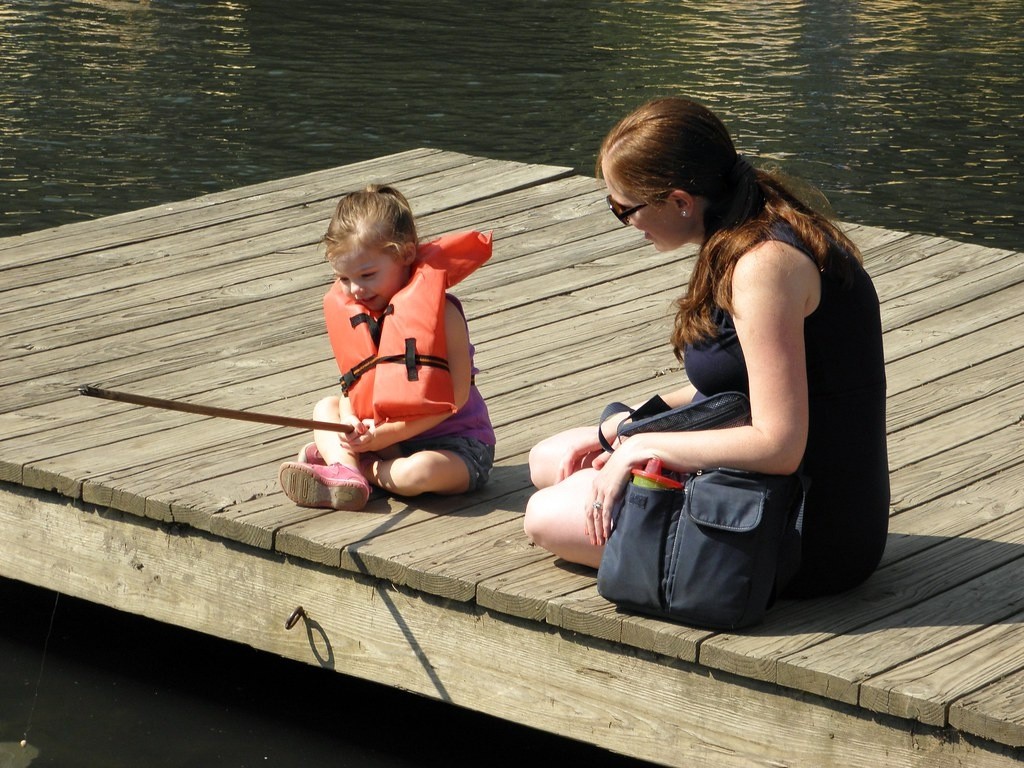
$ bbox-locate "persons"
[277,184,497,511]
[524,95,891,596]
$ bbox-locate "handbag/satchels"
[599,392,807,631]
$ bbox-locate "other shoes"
[279,443,372,512]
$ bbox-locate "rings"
[592,501,603,510]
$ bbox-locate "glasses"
[605,194,667,227]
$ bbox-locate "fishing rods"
[75,384,353,434]
